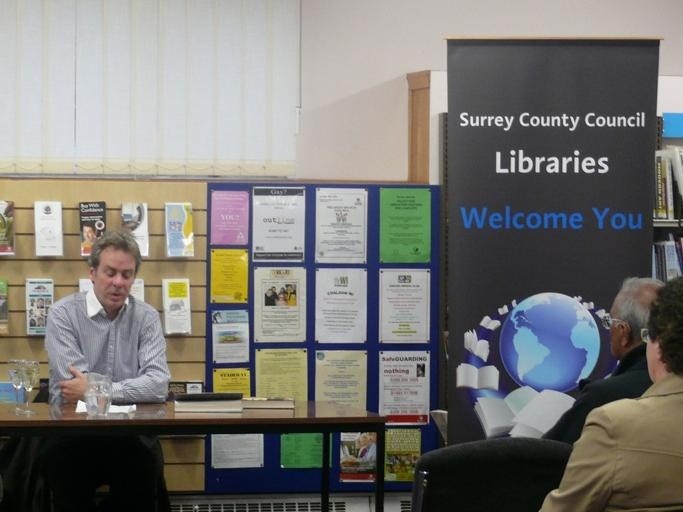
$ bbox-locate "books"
[173,392,243,414]
[473,385,539,439]
[652,232,683,276]
[653,144,683,220]
[242,396,296,410]
[653,276,679,282]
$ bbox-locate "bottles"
[84,374,112,418]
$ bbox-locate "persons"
[43,231,171,510]
[264,284,296,306]
[541,275,665,446]
[539,276,683,512]
[80,223,98,254]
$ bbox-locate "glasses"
[641,329,656,343]
[602,313,630,330]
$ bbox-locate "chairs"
[406,433,574,511]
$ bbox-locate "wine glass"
[23,361,39,414]
[8,359,25,414]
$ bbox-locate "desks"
[0,395,391,511]
